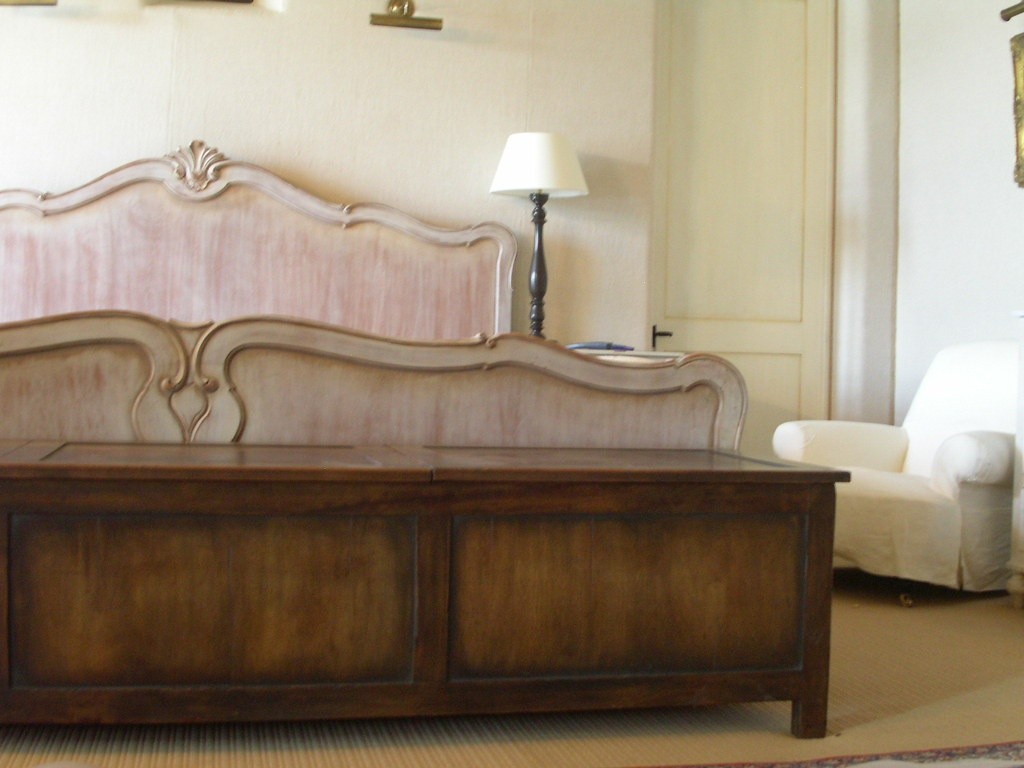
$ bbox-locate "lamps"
[489,129,589,339]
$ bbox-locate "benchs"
[0,437,858,739]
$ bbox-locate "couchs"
[773,340,1020,593]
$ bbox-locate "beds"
[1,145,754,447]
